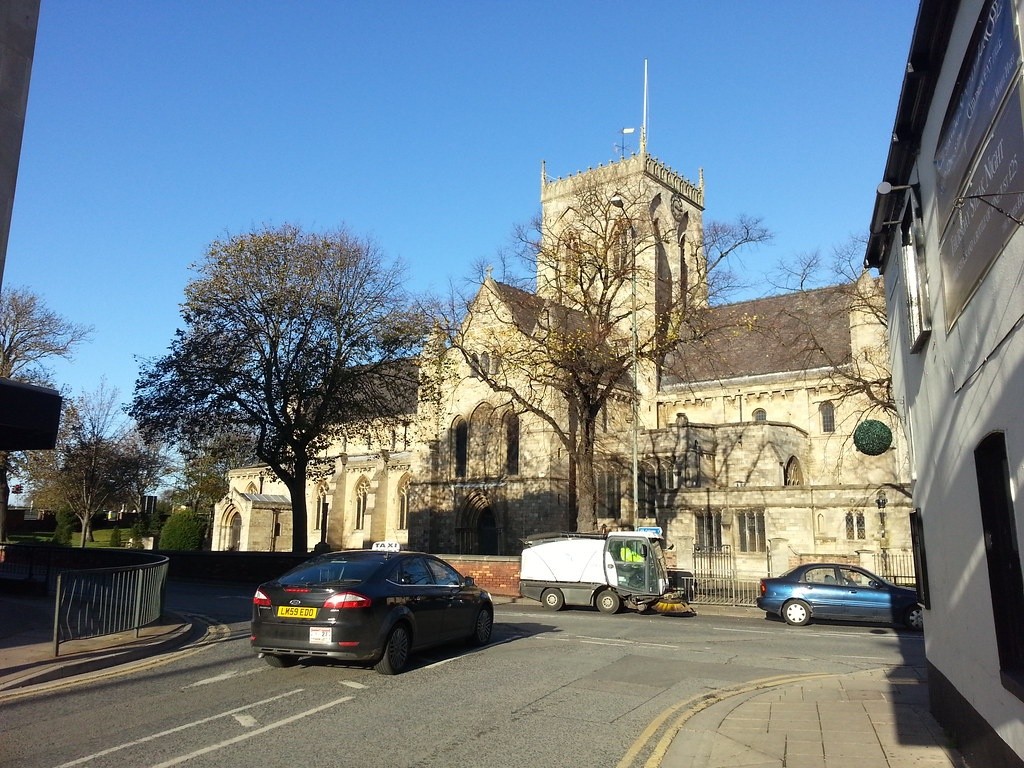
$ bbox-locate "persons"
[845,570,859,588]
[621,541,645,562]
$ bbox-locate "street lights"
[612,198,640,532]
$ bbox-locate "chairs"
[386,562,401,583]
[825,575,834,584]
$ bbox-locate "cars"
[756,561,925,633]
[250,541,495,677]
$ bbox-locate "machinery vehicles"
[519,525,696,618]
